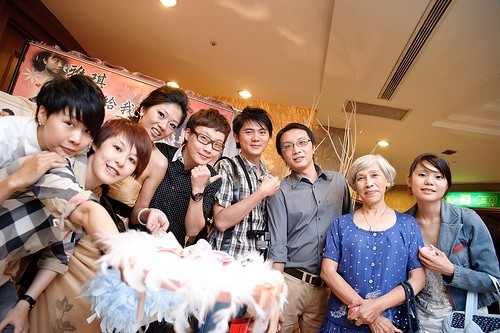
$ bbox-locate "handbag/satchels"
[392,281,424,333]
[443,274,500,333]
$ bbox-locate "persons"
[36,52,63,78]
[0,106,15,117]
[0,73,500,333]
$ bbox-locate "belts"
[284,267,327,287]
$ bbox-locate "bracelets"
[138,208,150,226]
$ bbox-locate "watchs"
[17,294,37,313]
[190,189,205,202]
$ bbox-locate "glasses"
[278,139,312,150]
[190,129,225,152]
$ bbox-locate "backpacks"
[185,155,241,253]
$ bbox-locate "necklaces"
[362,204,387,233]
